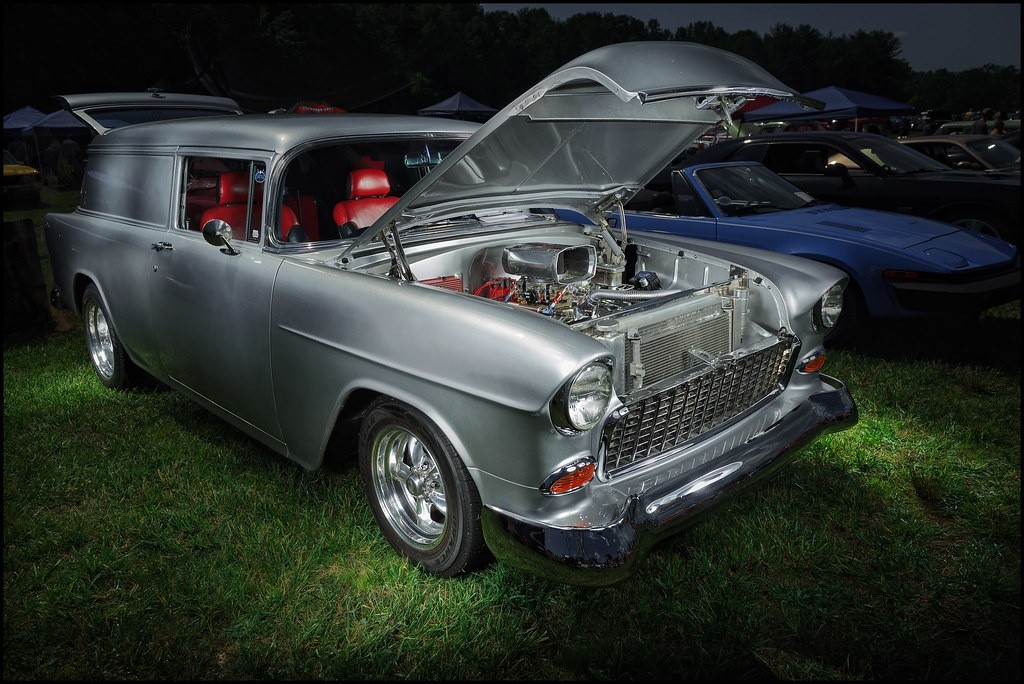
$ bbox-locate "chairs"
[332,169,401,240]
[200,171,311,242]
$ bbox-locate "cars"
[934,120,1020,151]
[826,135,1021,179]
[736,96,891,133]
[532,159,1023,345]
[3,39,860,584]
[685,117,831,149]
[2,149,43,197]
[644,132,1023,249]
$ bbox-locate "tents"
[3,106,48,131]
[416,91,500,120]
[22,108,132,182]
[742,85,916,134]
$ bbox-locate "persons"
[779,107,1020,140]
[6,133,84,177]
